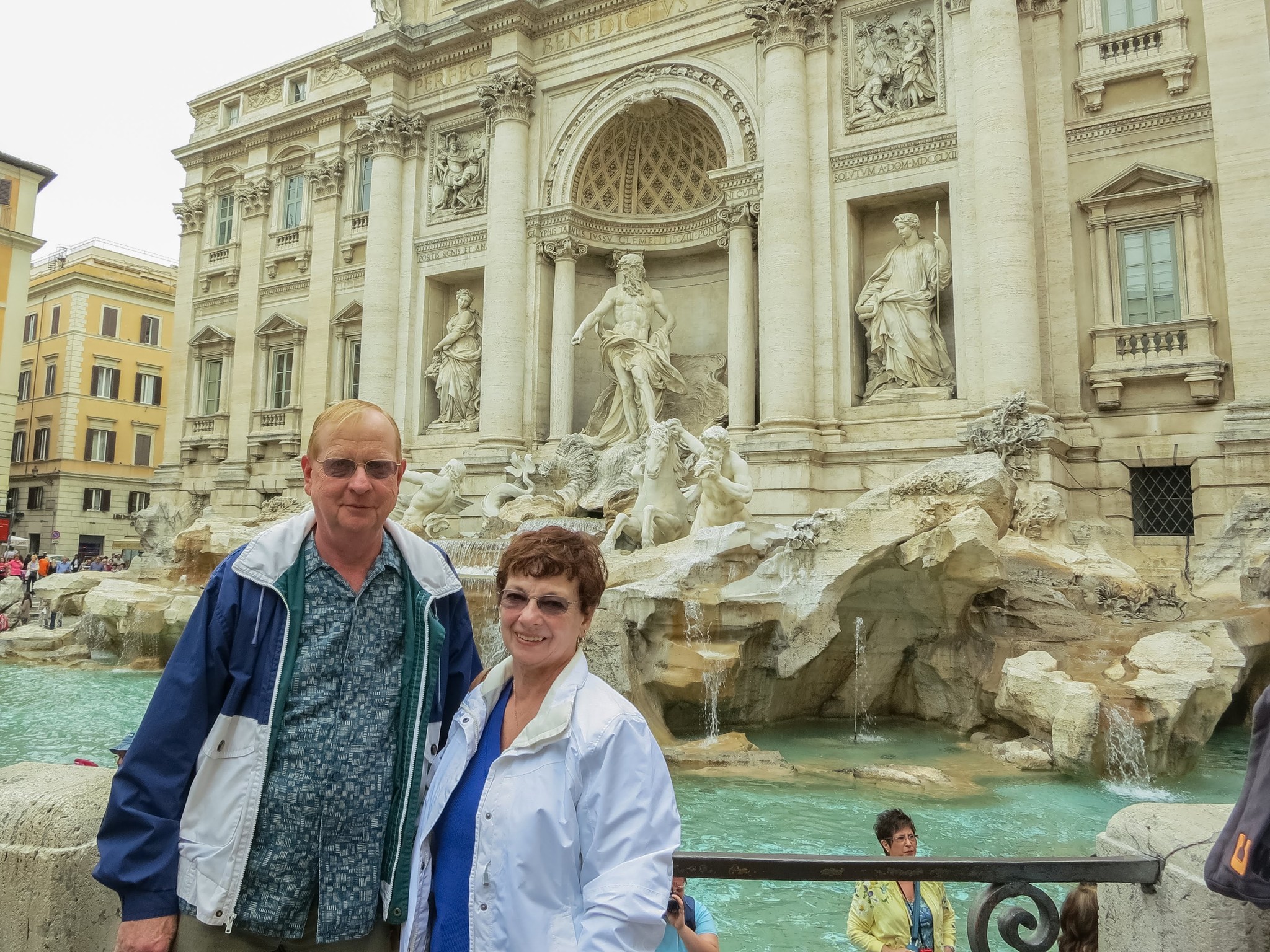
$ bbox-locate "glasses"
[103,561,107,562]
[884,835,919,843]
[671,880,687,894]
[314,457,401,480]
[496,588,583,617]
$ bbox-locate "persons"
[437,137,481,210]
[0,546,127,594]
[1057,854,1098,952]
[856,213,952,388]
[431,289,479,424]
[401,526,683,952]
[847,808,957,952]
[655,865,719,952]
[74,732,136,770]
[848,19,935,123]
[402,458,467,528]
[571,254,676,444]
[93,401,483,952]
[674,419,753,534]
[18,592,32,625]
[38,600,57,629]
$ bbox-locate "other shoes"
[32,591,35,595]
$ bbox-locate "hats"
[43,552,47,554]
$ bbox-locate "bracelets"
[944,946,955,952]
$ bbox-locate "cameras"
[667,891,680,914]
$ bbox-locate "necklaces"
[512,678,520,735]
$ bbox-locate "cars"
[37,554,71,565]
